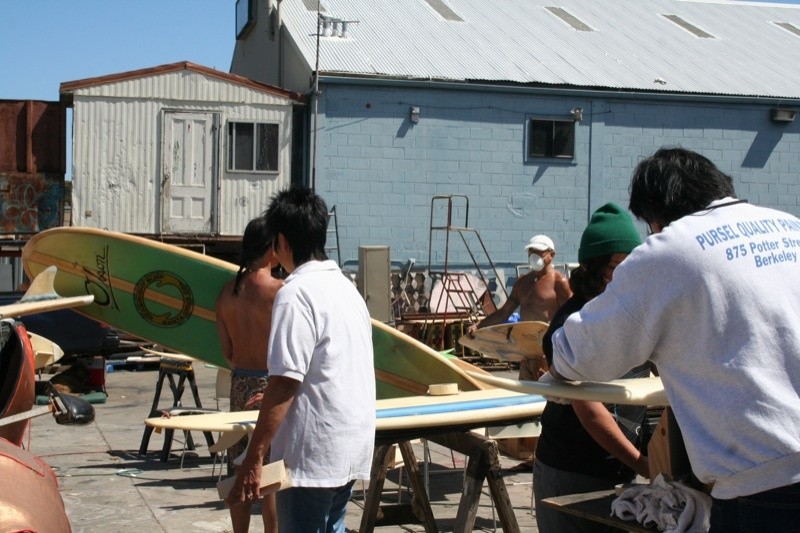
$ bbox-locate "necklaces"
[533,276,539,282]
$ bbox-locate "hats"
[525,235,555,251]
[577,201,643,262]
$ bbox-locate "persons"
[215,217,286,533]
[466,235,574,382]
[223,187,376,533]
[532,202,659,533]
[551,147,800,533]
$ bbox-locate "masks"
[527,253,546,272]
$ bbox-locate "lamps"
[570,107,584,122]
[410,106,420,124]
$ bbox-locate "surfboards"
[143,387,547,466]
[22,225,485,457]
[26,331,64,370]
[465,371,669,407]
[139,345,198,361]
[458,321,550,361]
[440,353,498,390]
[0,265,94,320]
